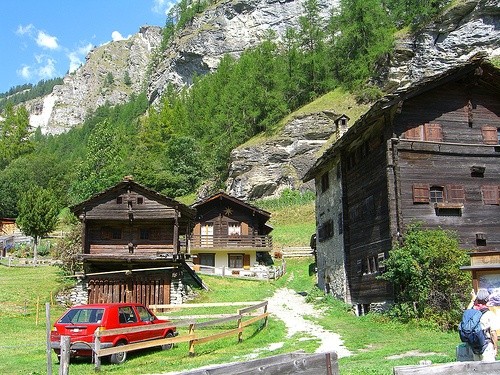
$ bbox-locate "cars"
[49,302,179,365]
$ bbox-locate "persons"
[467,288,497,361]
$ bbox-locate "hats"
[476,290,490,301]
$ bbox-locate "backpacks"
[458,306,492,354]
